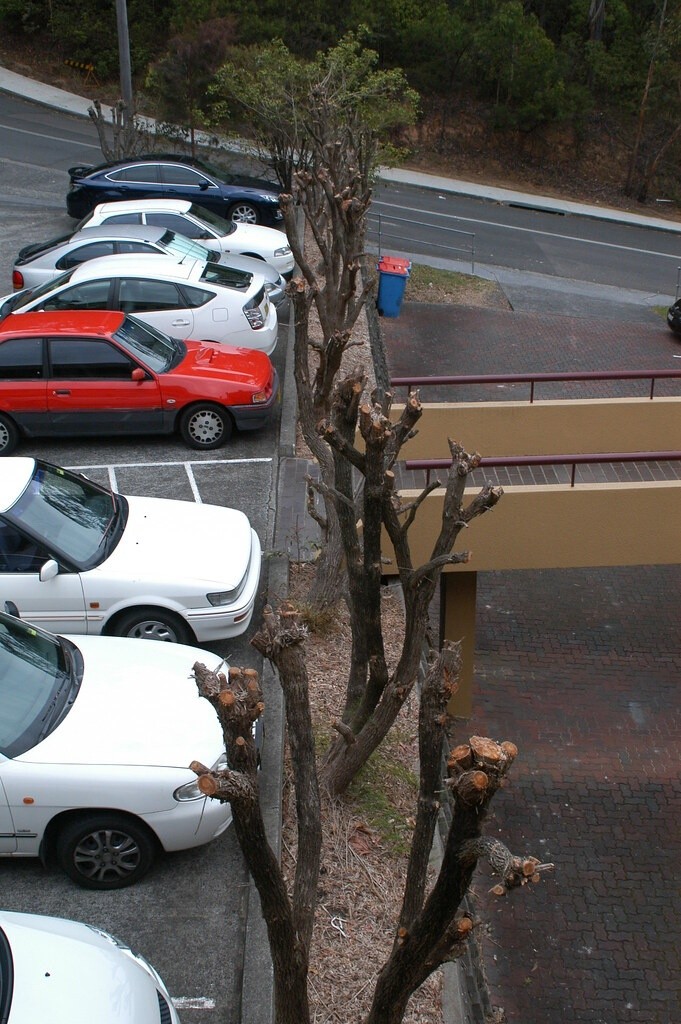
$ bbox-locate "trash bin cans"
[377,253,413,275]
[376,263,409,318]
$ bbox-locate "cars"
[77,198,296,276]
[13,223,288,308]
[0,909,182,1023]
[66,156,286,228]
[0,611,268,889]
[0,253,279,356]
[0,456,262,649]
[1,311,280,456]
[667,299,681,339]
